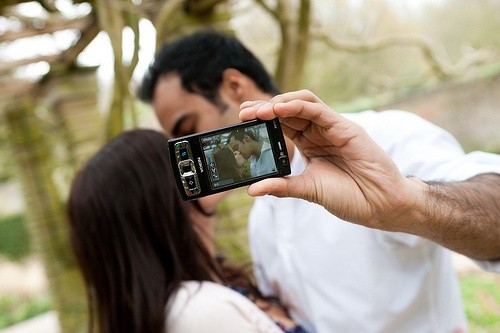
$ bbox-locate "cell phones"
[168,117,292,202]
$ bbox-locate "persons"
[63,125,306,333]
[133,27,500,333]
[226,128,278,177]
[214,144,252,184]
